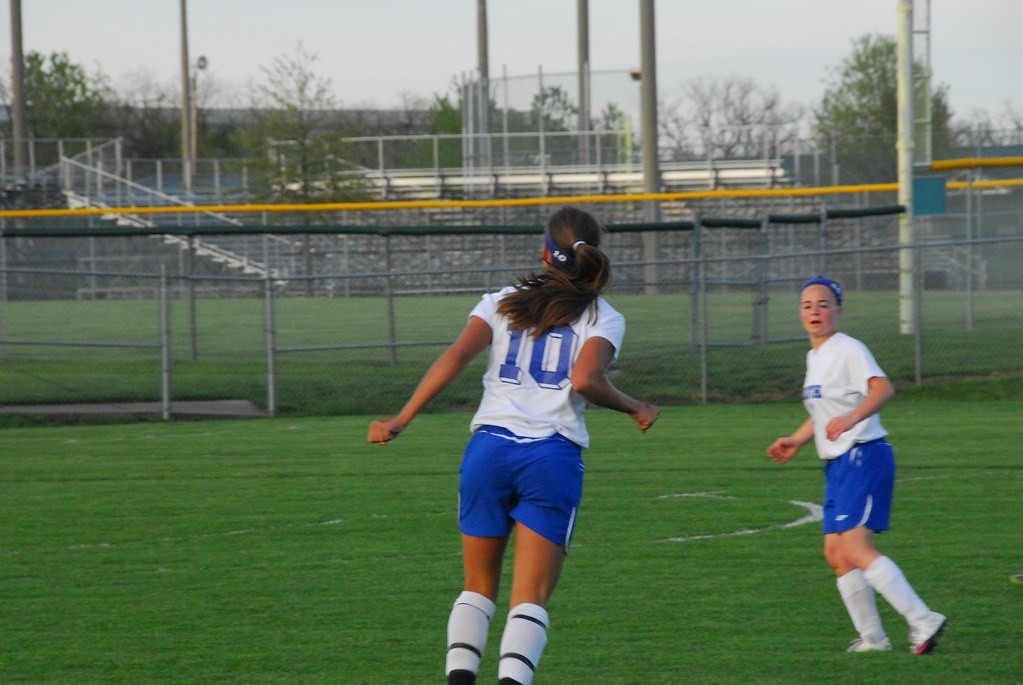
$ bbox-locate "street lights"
[192,55,209,185]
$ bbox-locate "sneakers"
[846,636,892,653]
[909,611,948,654]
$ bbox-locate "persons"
[766,275,948,655]
[368,207,661,685]
